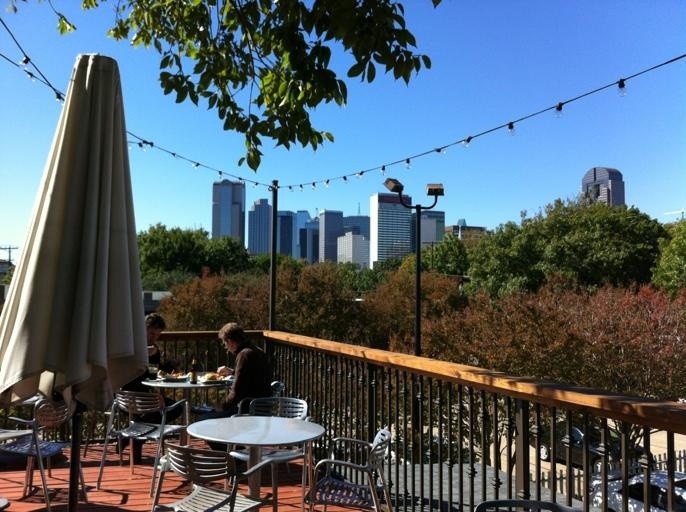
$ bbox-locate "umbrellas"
[0,53,150,512]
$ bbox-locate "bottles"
[190,355,197,384]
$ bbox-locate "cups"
[147,364,159,380]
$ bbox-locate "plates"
[165,377,188,381]
[201,380,224,384]
[146,378,166,382]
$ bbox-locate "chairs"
[0,398,86,506]
[310,429,391,512]
[151,443,262,512]
[474,500,585,512]
[227,397,308,493]
[270,381,284,398]
[96,391,186,496]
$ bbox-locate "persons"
[115,313,183,454]
[208,321,269,419]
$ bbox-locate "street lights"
[382,177,444,462]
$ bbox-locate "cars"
[530,417,654,479]
[591,470,685,512]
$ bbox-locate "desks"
[186,416,326,497]
